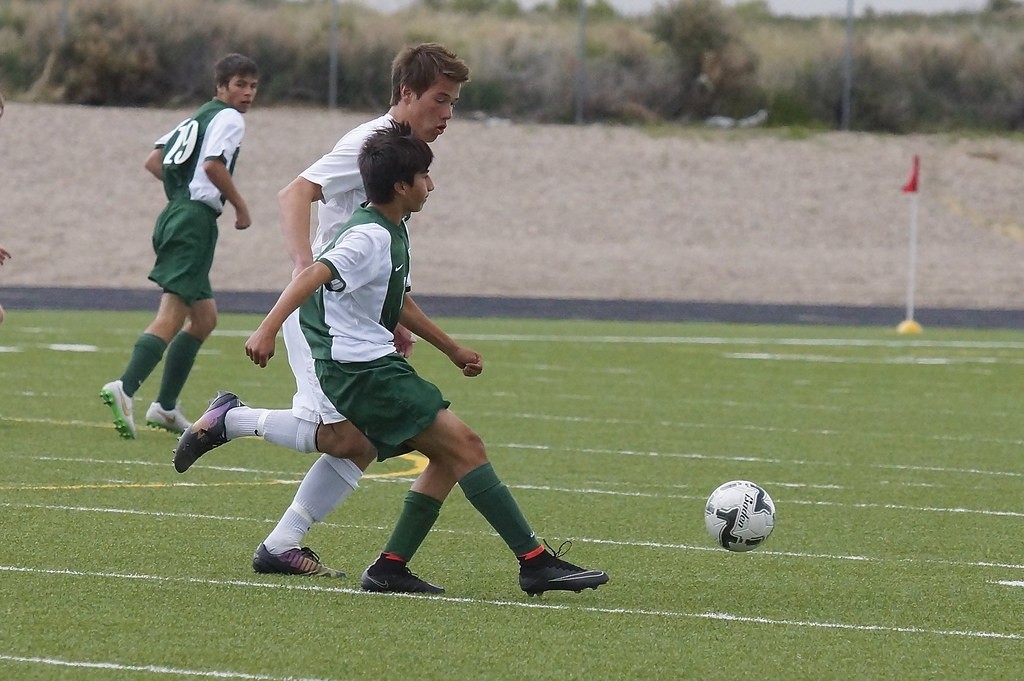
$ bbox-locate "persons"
[245,118,609,598]
[100,53,260,438]
[172,43,470,579]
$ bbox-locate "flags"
[902,157,919,192]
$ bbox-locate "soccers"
[701,478,778,553]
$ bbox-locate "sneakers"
[145,402,192,435]
[360,551,445,598]
[516,539,609,597]
[252,542,346,579]
[171,389,246,474]
[99,379,138,441]
